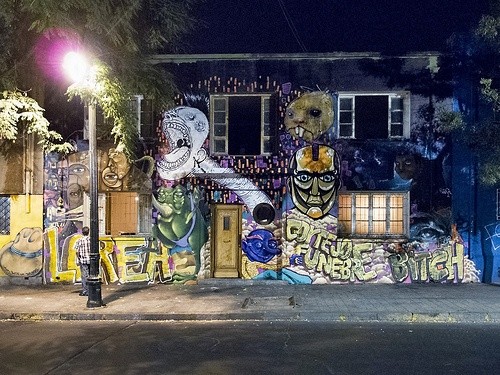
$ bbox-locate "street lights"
[62,50,105,309]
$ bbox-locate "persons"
[72,227,90,296]
